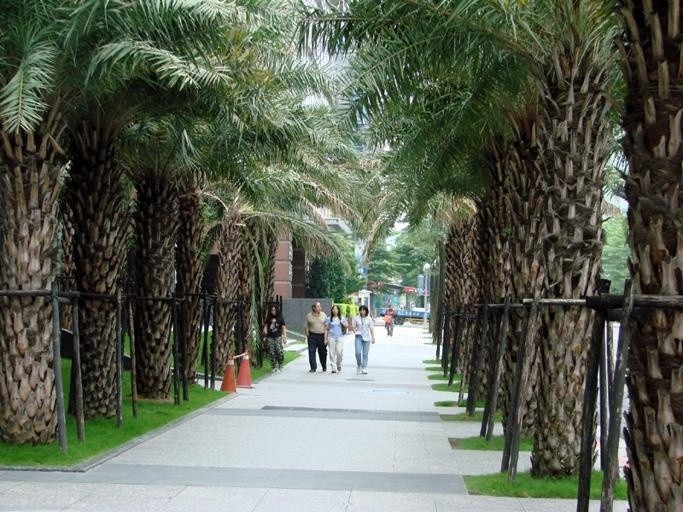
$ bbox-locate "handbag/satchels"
[341,323,346,335]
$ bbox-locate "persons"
[382,305,394,337]
[262,304,286,374]
[323,305,348,373]
[350,305,375,374]
[304,302,327,373]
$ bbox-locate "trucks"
[378,306,431,325]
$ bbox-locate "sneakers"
[323,368,326,372]
[271,367,281,373]
[308,369,316,373]
[356,368,362,374]
[331,368,337,374]
[337,367,342,371]
[362,368,368,374]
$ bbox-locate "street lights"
[423,262,431,330]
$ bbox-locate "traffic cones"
[218,350,237,392]
[234,349,253,389]
